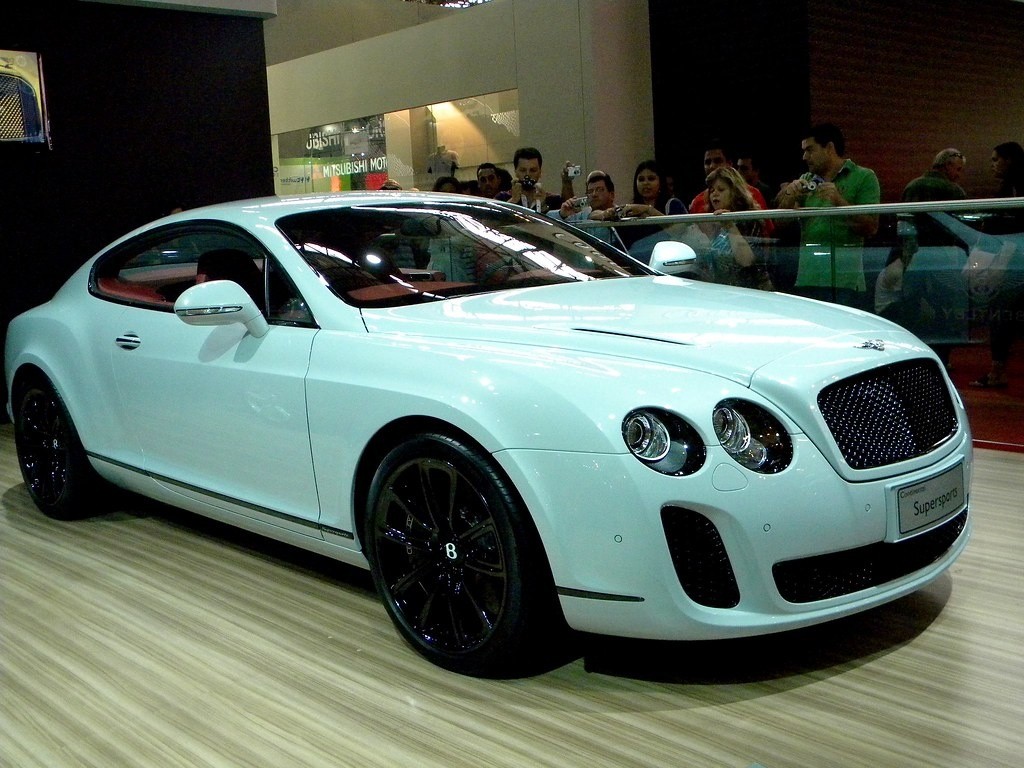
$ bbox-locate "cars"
[4,190,972,676]
[624,211,1024,387]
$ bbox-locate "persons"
[426,145,460,188]
[686,137,793,293]
[777,120,881,309]
[589,160,688,265]
[894,148,981,374]
[493,147,575,252]
[546,170,620,244]
[377,162,512,281]
[967,141,1024,389]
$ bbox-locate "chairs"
[197,250,266,318]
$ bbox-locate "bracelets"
[647,204,654,214]
[559,210,568,220]
[785,189,791,195]
[725,223,732,230]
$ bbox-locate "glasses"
[949,153,964,159]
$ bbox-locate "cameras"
[802,181,820,193]
[514,175,537,193]
[567,165,581,177]
[572,197,589,210]
